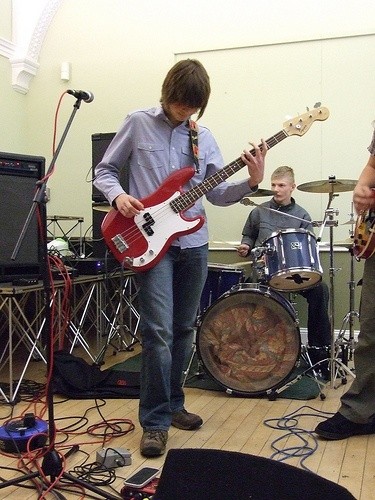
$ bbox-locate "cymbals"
[92,205,114,212]
[296,179,360,193]
[339,220,358,224]
[243,188,280,197]
[324,237,357,248]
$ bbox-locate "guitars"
[99,101,331,274]
[352,188,375,261]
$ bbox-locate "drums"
[196,282,302,394]
[195,262,245,326]
[261,228,324,292]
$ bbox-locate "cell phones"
[123,467,159,489]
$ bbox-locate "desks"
[0,270,142,404]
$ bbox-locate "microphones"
[67,89,94,103]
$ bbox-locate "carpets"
[112,348,330,401]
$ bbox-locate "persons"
[93,58,268,458]
[313,130,375,440]
[238,166,334,382]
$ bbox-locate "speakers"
[91,201,115,259]
[91,133,129,201]
[0,152,45,282]
[153,448,358,500]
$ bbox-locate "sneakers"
[140,429,168,456]
[315,412,375,440]
[172,409,202,429]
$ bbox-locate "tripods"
[269,195,356,399]
[0,104,123,500]
